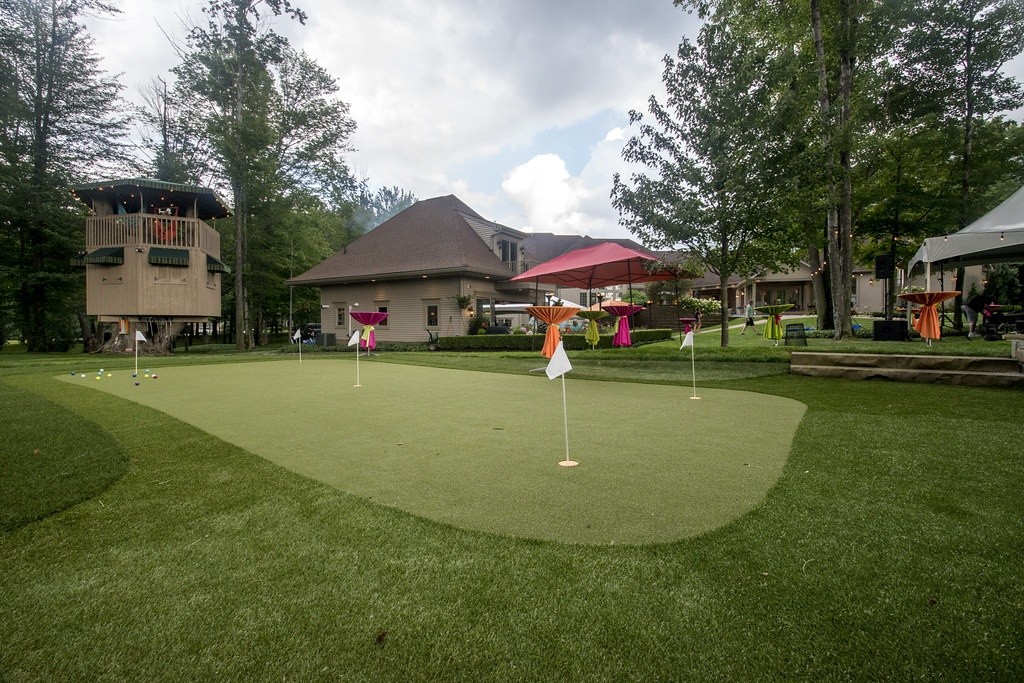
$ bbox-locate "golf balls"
[71,372,75,375]
[135,382,140,386]
[96,377,101,380]
[152,374,155,377]
[107,374,112,377]
[144,374,149,378]
[81,374,86,378]
[145,370,149,373]
[97,371,102,376]
[154,376,157,379]
[100,369,104,372]
[132,374,137,377]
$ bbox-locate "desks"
[985,313,1024,323]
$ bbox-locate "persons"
[960,295,996,338]
[529,314,535,325]
[694,307,702,333]
[570,319,582,332]
[740,300,761,335]
[503,319,508,326]
[565,328,571,334]
[301,326,313,343]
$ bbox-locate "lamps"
[82,250,88,255]
[521,247,525,254]
[869,278,873,285]
[135,247,145,252]
[468,307,474,317]
[498,241,503,249]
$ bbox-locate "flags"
[136,330,147,342]
[546,342,572,380]
[681,332,693,350]
[348,331,359,346]
[293,328,301,340]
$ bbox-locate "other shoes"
[968,332,981,337]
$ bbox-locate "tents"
[907,185,1024,343]
[510,242,702,352]
